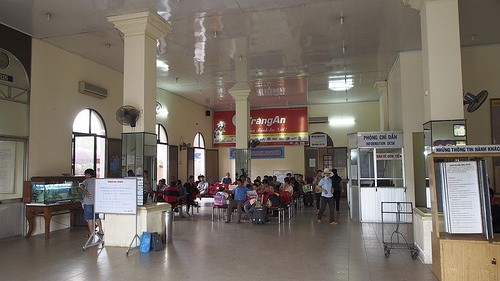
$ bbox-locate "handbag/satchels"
[214,195,226,207]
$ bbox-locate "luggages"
[252,193,267,224]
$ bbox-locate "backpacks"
[267,192,288,209]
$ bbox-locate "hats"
[321,168,334,177]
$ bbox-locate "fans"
[249,138,260,149]
[180,142,191,151]
[115,105,140,127]
[464,89,488,113]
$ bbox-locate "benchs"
[151,194,304,224]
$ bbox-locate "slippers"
[95,232,104,235]
[86,234,93,238]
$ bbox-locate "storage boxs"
[25,180,81,206]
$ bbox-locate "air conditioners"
[78,80,107,100]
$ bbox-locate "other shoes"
[317,218,322,223]
[330,222,338,225]
[225,220,230,223]
[236,220,240,224]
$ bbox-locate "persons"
[214,169,307,224]
[128,170,135,176]
[225,179,250,224]
[331,169,342,214]
[313,170,324,214]
[80,169,104,237]
[156,175,209,216]
[316,168,338,224]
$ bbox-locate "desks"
[24,202,84,239]
